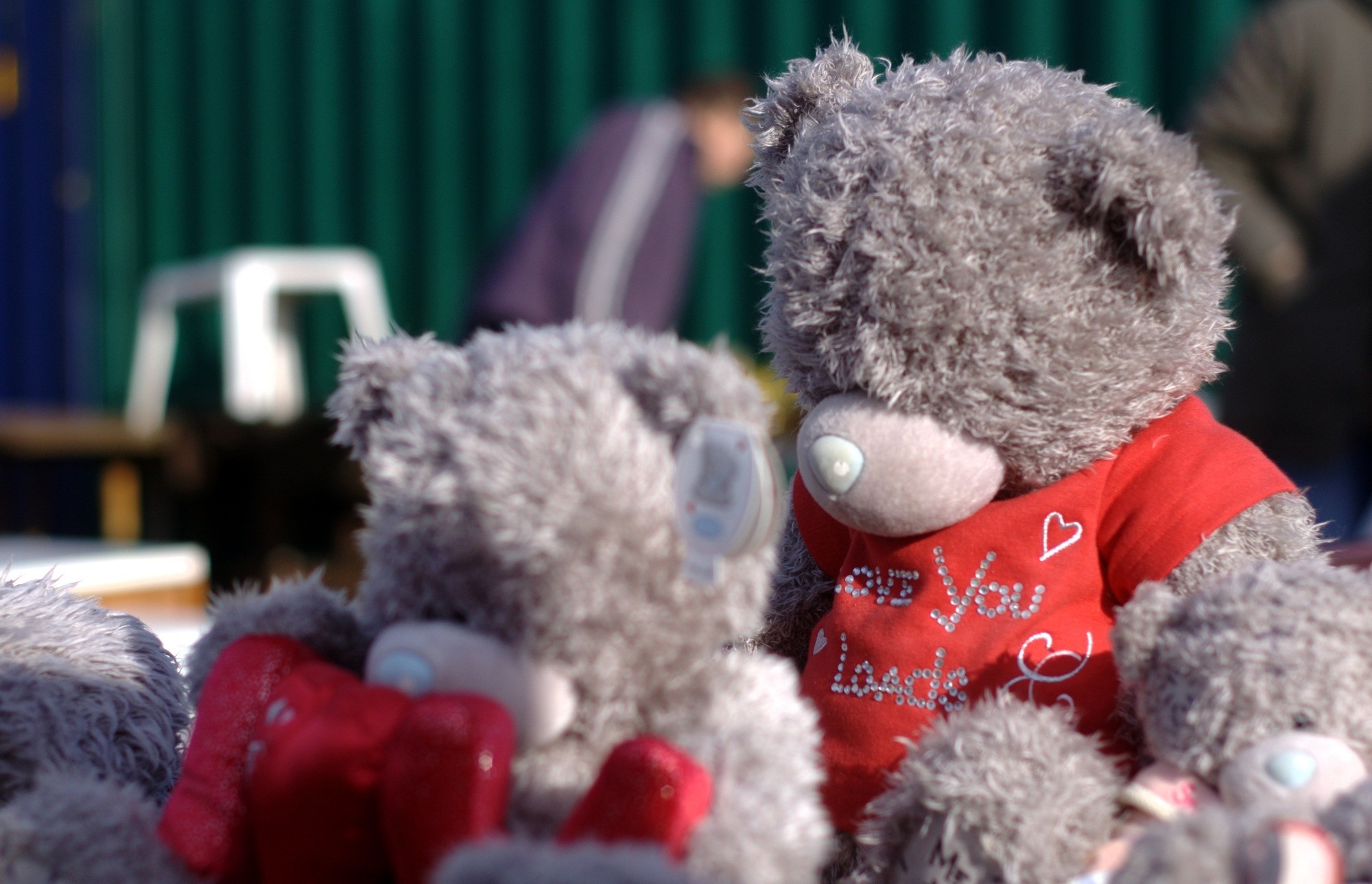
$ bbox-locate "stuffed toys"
[0,306,832,884]
[736,23,1370,881]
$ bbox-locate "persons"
[454,78,762,342]
[1170,0,1372,553]
